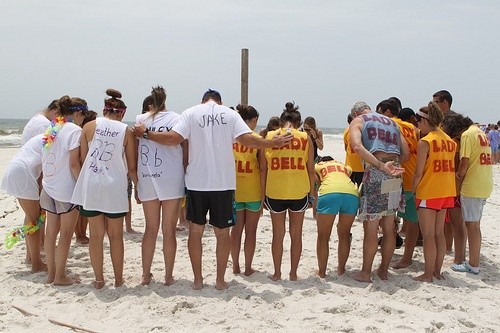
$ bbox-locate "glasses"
[435,101,442,104]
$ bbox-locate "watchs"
[143,126,152,140]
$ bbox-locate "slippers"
[451,261,481,275]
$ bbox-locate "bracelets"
[314,136,318,140]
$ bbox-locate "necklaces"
[42,115,76,147]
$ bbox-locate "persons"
[134,87,189,285]
[443,111,493,274]
[258,116,280,139]
[45,95,87,286]
[376,101,423,270]
[260,102,318,281]
[348,100,410,283]
[311,156,362,277]
[67,87,138,290]
[74,102,323,247]
[343,113,366,214]
[230,104,262,278]
[128,86,295,289]
[299,116,324,207]
[375,90,500,263]
[1,126,48,273]
[19,100,63,245]
[409,101,456,282]
